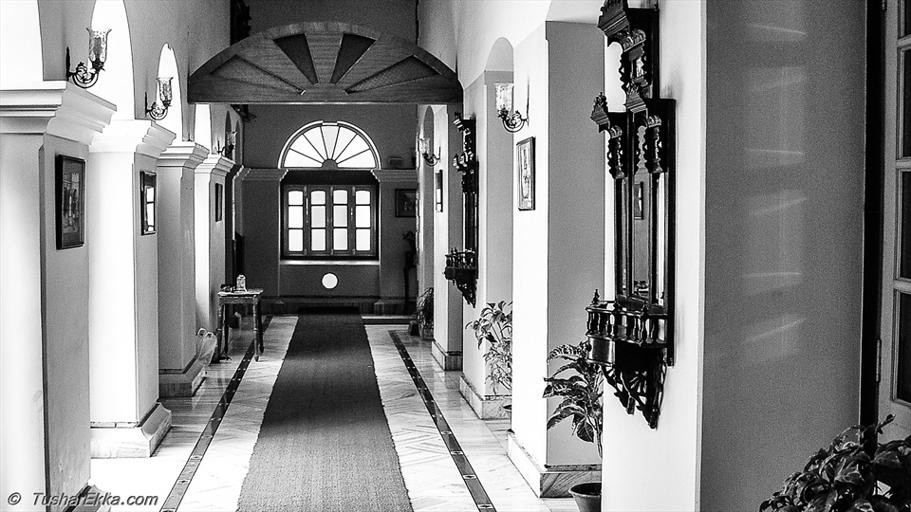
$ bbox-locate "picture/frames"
[395,188,416,218]
[516,136,534,212]
[139,170,157,235]
[215,183,223,222]
[55,154,85,249]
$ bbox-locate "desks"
[212,286,264,362]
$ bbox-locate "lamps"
[65,27,112,89]
[218,130,237,156]
[416,138,440,166]
[144,76,174,120]
[492,82,529,132]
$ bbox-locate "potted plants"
[465,300,512,425]
[540,339,604,512]
